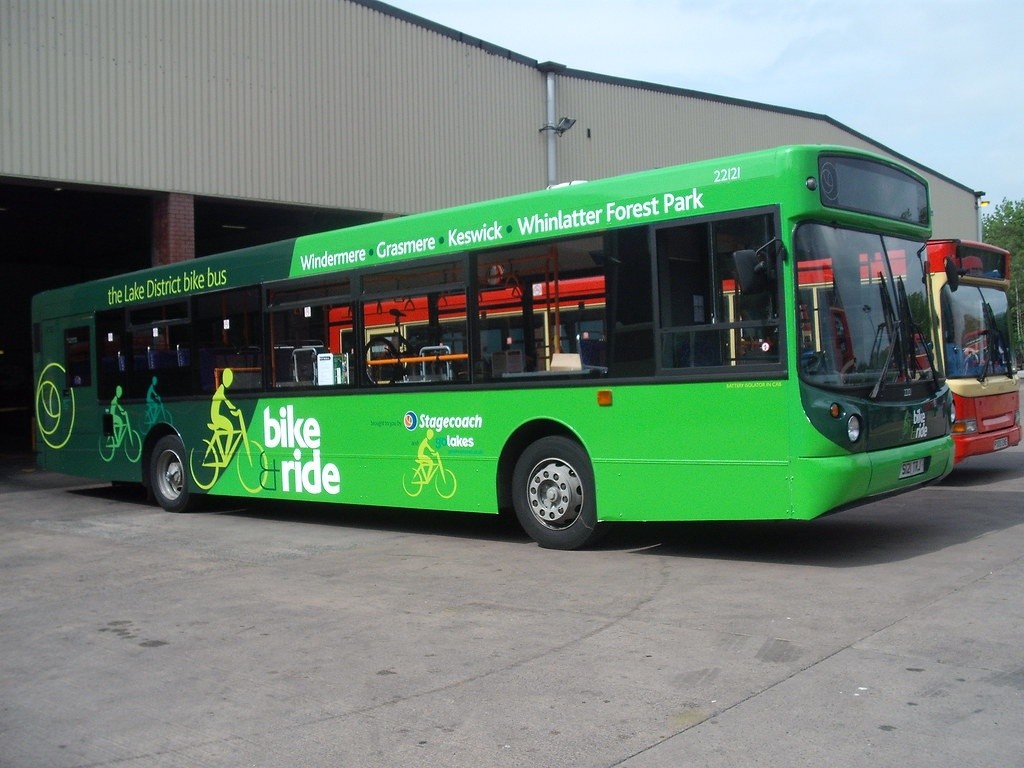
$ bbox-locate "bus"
[330,237,1020,464]
[27,145,957,545]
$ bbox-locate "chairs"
[106,329,609,400]
